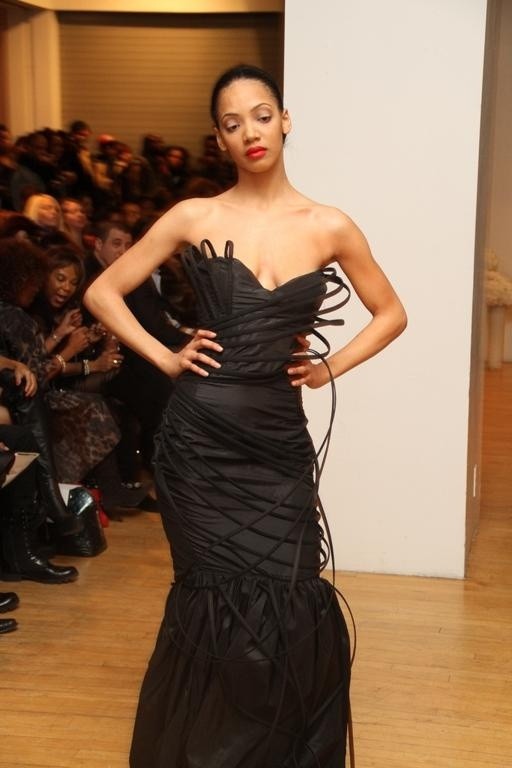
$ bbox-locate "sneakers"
[117,482,164,514]
[0,618,18,634]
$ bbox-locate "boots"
[1,499,79,587]
[0,367,76,524]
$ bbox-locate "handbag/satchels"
[43,487,109,559]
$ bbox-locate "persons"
[79,62,414,767]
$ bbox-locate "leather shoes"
[0,592,20,614]
